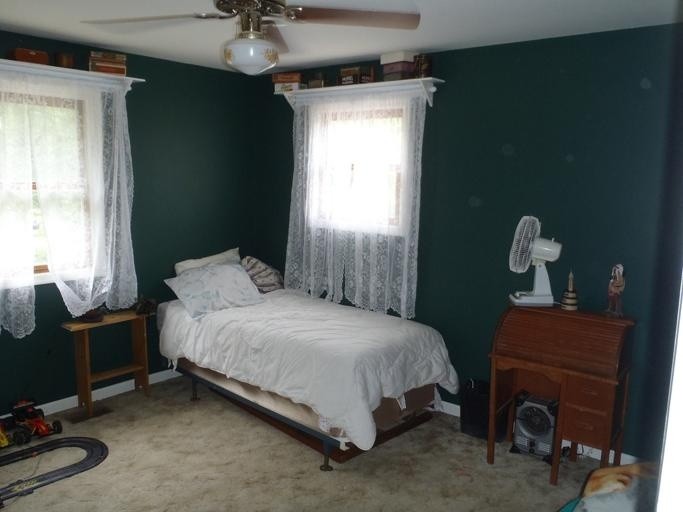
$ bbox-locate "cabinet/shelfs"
[62,312,154,417]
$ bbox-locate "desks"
[486,306,640,486]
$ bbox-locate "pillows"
[171,247,242,273]
[242,256,284,294]
[164,263,265,319]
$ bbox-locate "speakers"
[509,389,560,459]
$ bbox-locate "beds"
[154,245,460,471]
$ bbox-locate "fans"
[76,1,421,53]
[508,216,562,306]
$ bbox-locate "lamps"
[223,12,279,77]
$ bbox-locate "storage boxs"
[12,48,48,65]
[86,57,128,79]
